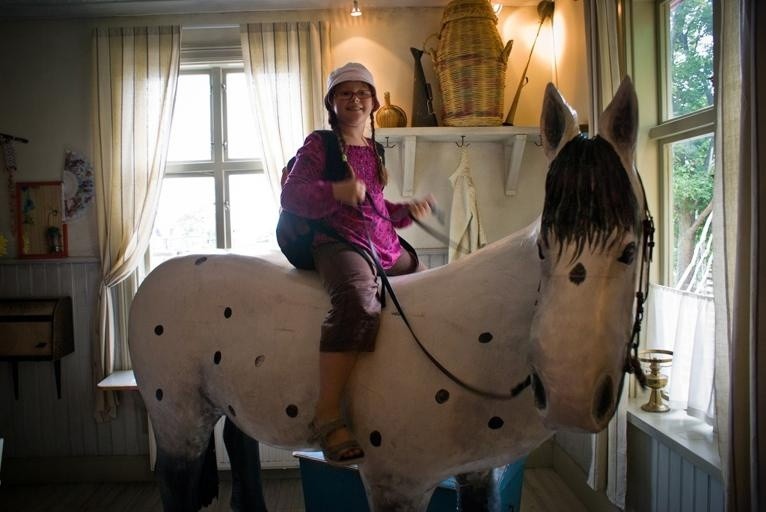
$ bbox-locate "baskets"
[422,0,513,127]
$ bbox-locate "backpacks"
[275,130,385,270]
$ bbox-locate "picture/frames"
[16,181,70,259]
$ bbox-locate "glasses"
[338,89,372,101]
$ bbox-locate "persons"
[280,62,439,468]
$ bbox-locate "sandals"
[308,413,365,461]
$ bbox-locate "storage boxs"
[291,447,527,512]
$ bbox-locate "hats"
[324,64,380,114]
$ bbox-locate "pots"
[376,90,406,128]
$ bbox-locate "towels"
[446,146,489,266]
